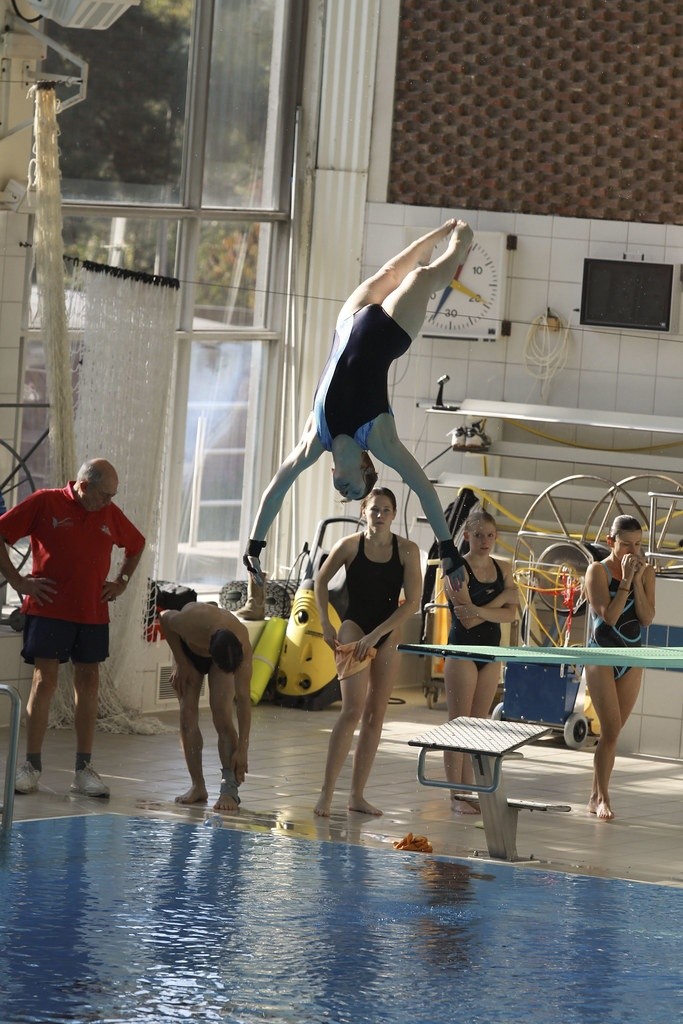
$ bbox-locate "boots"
[235,572,267,621]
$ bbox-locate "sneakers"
[15,761,42,793]
[70,760,110,798]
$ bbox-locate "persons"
[159,602,254,808]
[0,458,145,797]
[313,487,422,817]
[243,219,474,592]
[586,515,656,818]
[443,510,522,814]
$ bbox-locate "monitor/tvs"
[580,258,674,332]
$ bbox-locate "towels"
[332,639,377,681]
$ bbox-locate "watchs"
[118,574,129,582]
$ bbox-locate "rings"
[109,596,111,598]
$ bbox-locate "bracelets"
[618,588,629,591]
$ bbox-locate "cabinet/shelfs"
[415,398,682,548]
